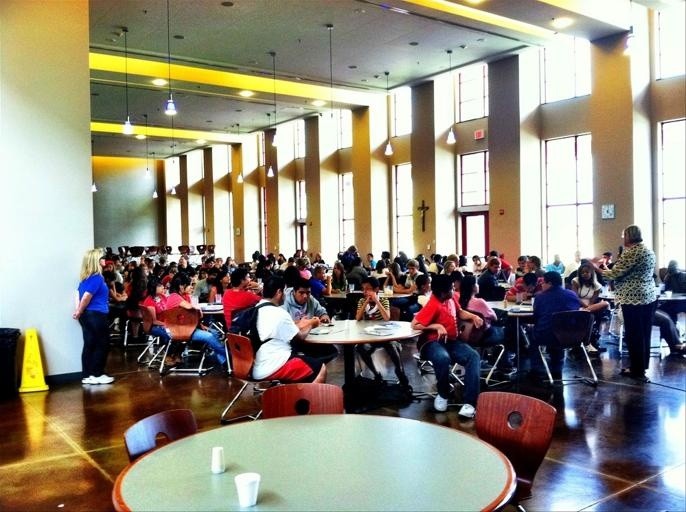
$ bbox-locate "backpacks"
[229,303,275,353]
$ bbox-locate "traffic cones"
[16,329,52,393]
[19,391,52,449]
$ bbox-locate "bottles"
[343,319,350,331]
[209,447,225,475]
[503,300,508,309]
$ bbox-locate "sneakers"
[433,393,447,411]
[82,375,114,385]
[458,402,476,418]
[619,366,646,376]
[670,343,685,356]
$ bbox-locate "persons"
[651,309,686,354]
[101,246,618,418]
[588,225,656,378]
[661,261,686,339]
[72,248,115,384]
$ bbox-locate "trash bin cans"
[0,328,19,402]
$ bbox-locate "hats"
[405,259,421,270]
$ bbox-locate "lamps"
[90,0,459,201]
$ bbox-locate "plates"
[364,326,392,336]
[200,304,223,312]
[310,326,332,335]
[374,323,401,329]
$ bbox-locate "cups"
[655,283,673,298]
[532,297,536,307]
[516,293,524,304]
[214,293,221,303]
[349,284,355,293]
[602,287,609,296]
[383,285,389,296]
[387,285,395,297]
[510,273,516,282]
[233,472,261,509]
[257,278,262,284]
[191,296,198,306]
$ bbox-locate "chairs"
[476,393,557,509]
[261,384,342,420]
[123,408,198,464]
[114,273,686,387]
[221,334,280,423]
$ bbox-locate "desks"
[305,320,422,408]
[113,414,516,510]
